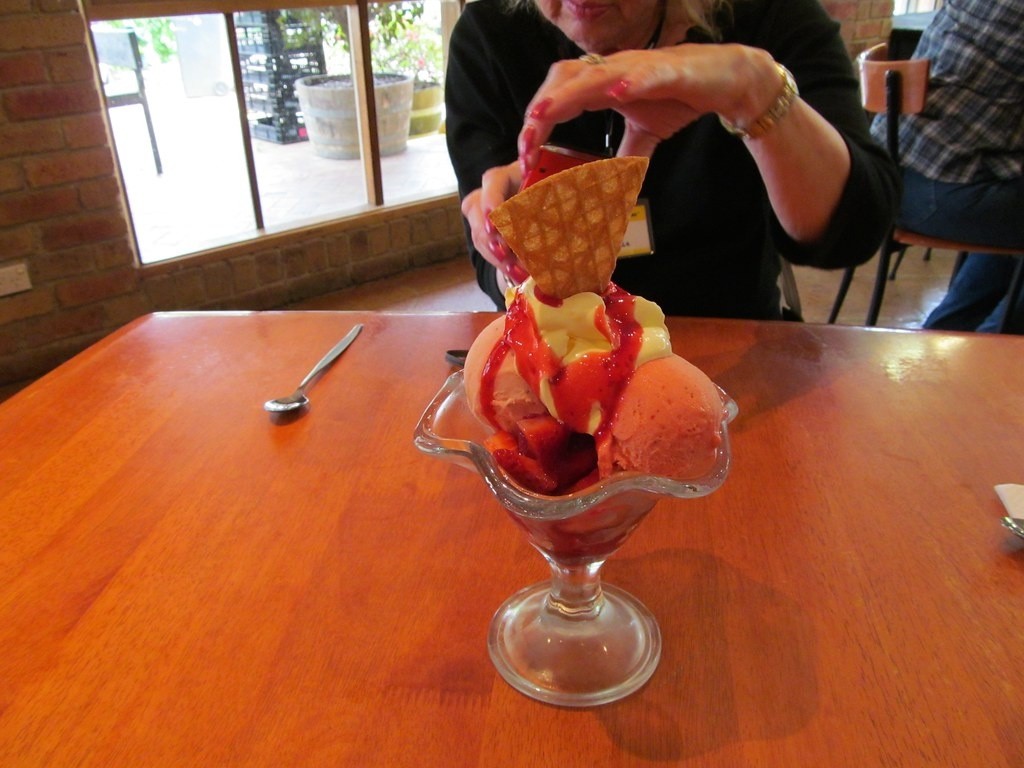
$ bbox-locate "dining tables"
[0,308,1024,768]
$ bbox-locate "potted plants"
[279,0,430,159]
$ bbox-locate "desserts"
[462,147,726,545]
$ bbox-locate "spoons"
[263,324,363,412]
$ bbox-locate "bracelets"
[718,62,798,138]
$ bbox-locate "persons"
[445,0,898,318]
[869,0,1024,334]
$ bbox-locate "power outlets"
[0,265,32,297]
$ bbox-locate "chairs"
[828,26,1023,333]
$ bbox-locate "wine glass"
[413,370,741,707]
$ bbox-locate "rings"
[580,53,607,63]
